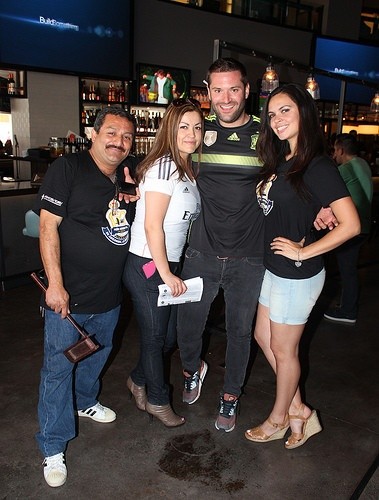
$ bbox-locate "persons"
[244,85,361,450]
[117,58,340,432]
[322,132,373,324]
[37,106,140,486]
[123,99,202,428]
[140,70,178,105]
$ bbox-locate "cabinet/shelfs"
[78,76,211,157]
[315,99,379,125]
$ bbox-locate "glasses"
[170,98,201,109]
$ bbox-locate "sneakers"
[75,402,117,423]
[214,390,241,433]
[42,452,67,488]
[182,360,208,405]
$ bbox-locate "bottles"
[8,73,15,95]
[49,80,210,157]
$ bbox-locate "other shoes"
[324,308,357,323]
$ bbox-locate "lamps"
[305,72,320,100]
[261,61,279,93]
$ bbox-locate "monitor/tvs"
[309,33,379,107]
[136,62,192,107]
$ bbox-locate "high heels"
[285,403,322,450]
[244,413,290,442]
[145,401,185,428]
[126,376,148,411]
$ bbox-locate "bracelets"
[294,247,303,268]
